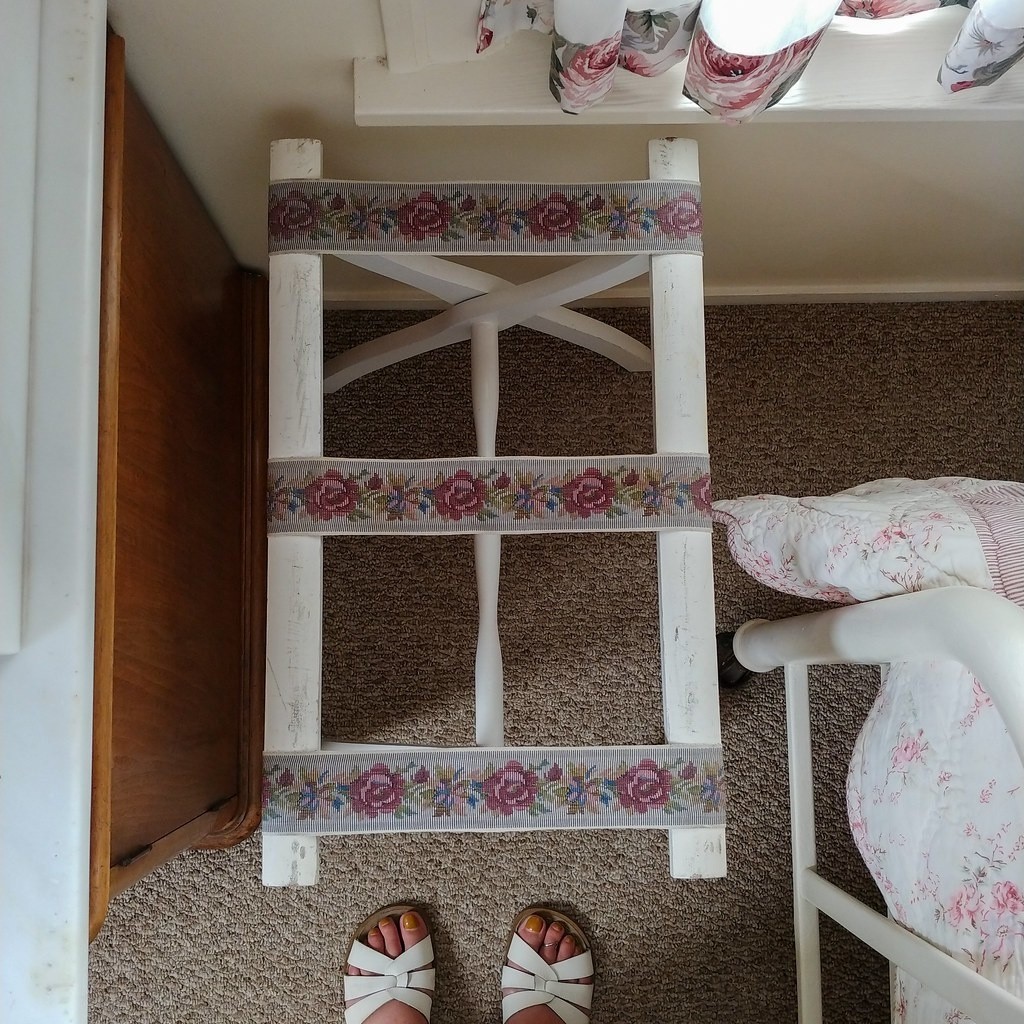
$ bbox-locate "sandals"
[500,907,593,1024]
[343,905,435,1024]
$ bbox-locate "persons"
[342,903,596,1024]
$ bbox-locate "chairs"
[713,479,1024,1024]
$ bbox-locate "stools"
[268,138,730,888]
